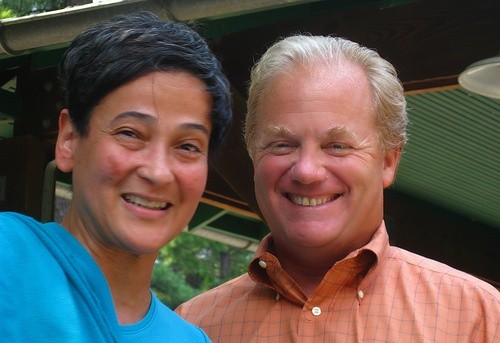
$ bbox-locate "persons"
[173,34,500,343]
[0,10,234,343]
